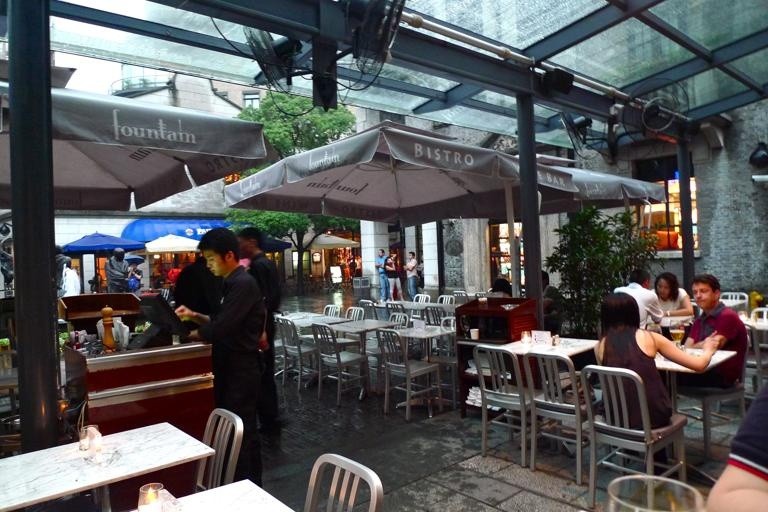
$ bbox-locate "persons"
[541,270,562,336]
[104,247,143,293]
[55,246,81,297]
[171,227,283,487]
[706,381,768,510]
[593,268,748,479]
[376,248,418,303]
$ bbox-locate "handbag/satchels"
[128,276,140,289]
[548,371,596,406]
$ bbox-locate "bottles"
[73,331,81,351]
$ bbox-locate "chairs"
[0,381,87,459]
[195,407,247,493]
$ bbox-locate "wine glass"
[669,319,685,350]
[646,324,662,335]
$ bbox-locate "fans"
[321,0,405,112]
[242,26,314,95]
[608,76,690,166]
[560,110,608,160]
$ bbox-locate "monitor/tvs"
[139,292,187,335]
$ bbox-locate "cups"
[520,330,531,344]
[78,424,103,467]
[737,310,748,323]
[470,328,479,340]
[137,482,164,512]
[605,474,705,512]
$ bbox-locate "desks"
[129,476,299,512]
[0,422,214,512]
